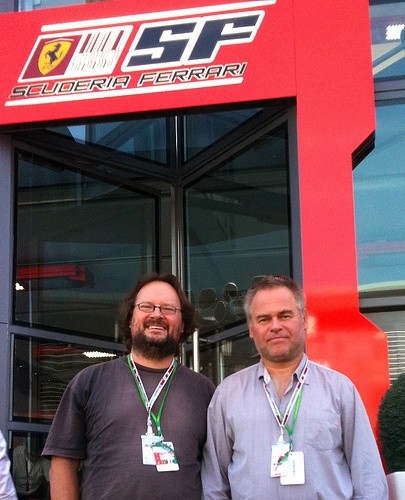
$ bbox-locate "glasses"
[132,302,186,316]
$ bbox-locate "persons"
[201,275,389,500]
[0,430,18,500]
[41,274,214,500]
[13,435,51,498]
